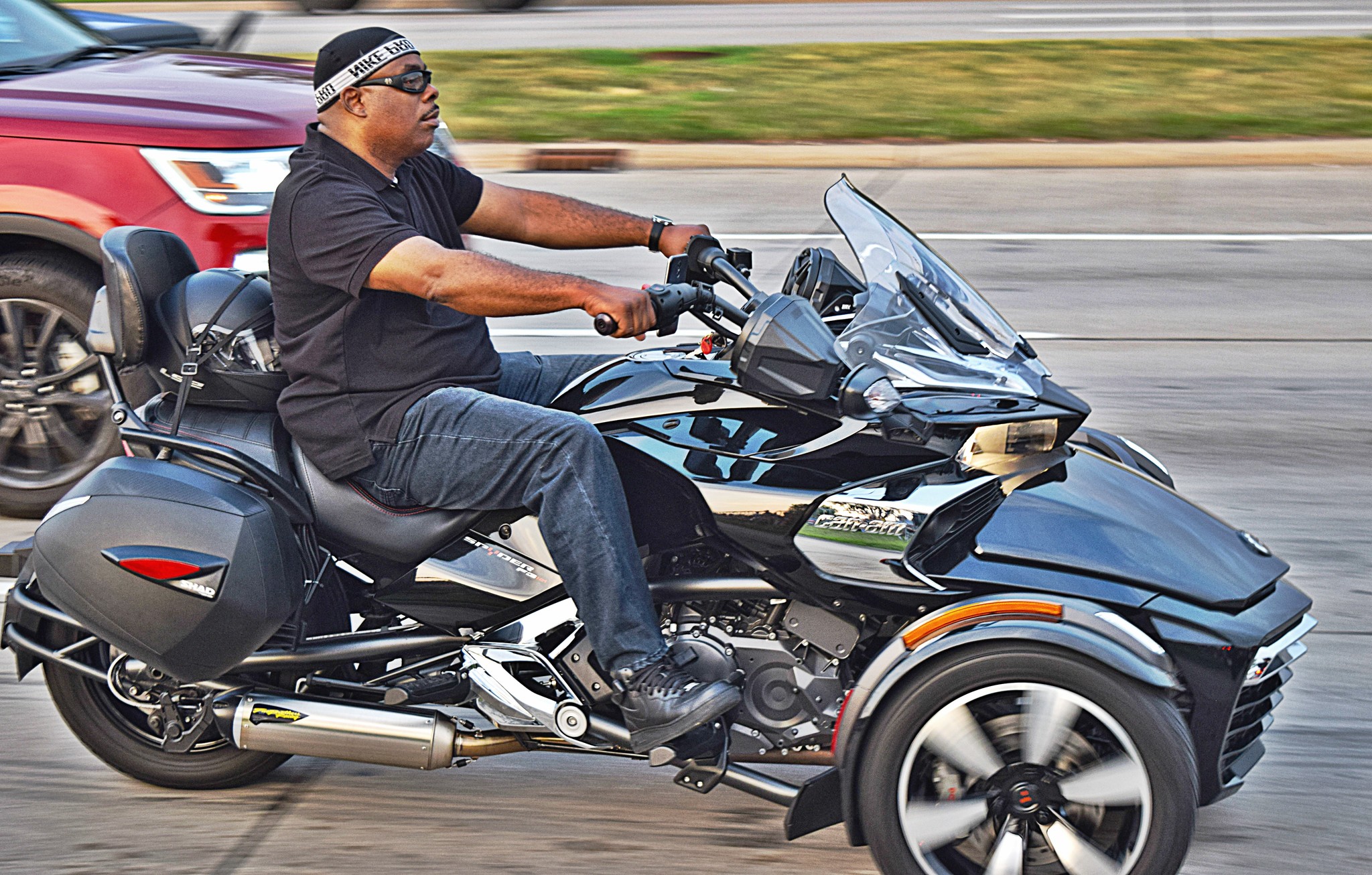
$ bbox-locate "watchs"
[647,214,673,253]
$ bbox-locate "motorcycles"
[0,169,1319,875]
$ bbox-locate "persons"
[268,26,744,755]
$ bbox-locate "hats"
[313,27,421,115]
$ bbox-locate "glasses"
[352,70,433,94]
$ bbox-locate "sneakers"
[611,654,743,755]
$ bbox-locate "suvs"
[0,0,467,520]
